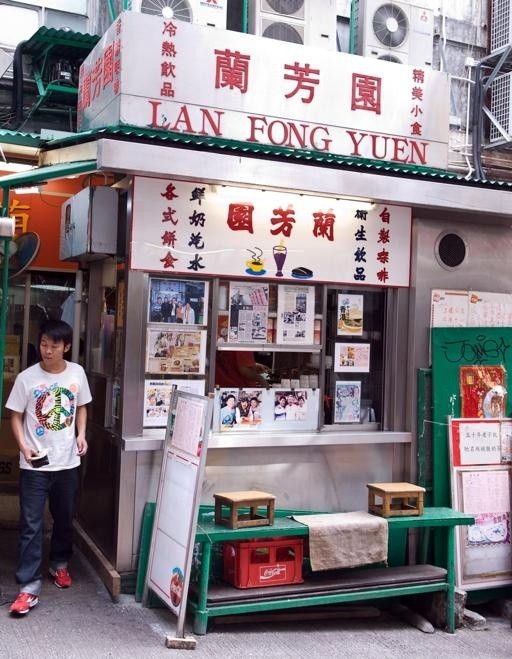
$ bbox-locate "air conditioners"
[133,0,228,30]
[349,1,434,69]
[245,0,339,49]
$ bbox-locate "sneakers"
[47,564,72,589]
[6,590,40,617]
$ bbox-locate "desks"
[192,507,478,640]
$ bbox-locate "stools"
[366,481,427,518]
[213,492,277,531]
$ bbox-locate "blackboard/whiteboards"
[446,415,512,592]
[141,384,215,619]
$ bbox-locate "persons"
[340,347,355,367]
[219,384,359,428]
[0,318,95,617]
[13,322,37,373]
[207,276,274,390]
[216,283,308,345]
[146,293,204,418]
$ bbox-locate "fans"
[0,232,41,281]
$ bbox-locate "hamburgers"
[337,308,363,334]
